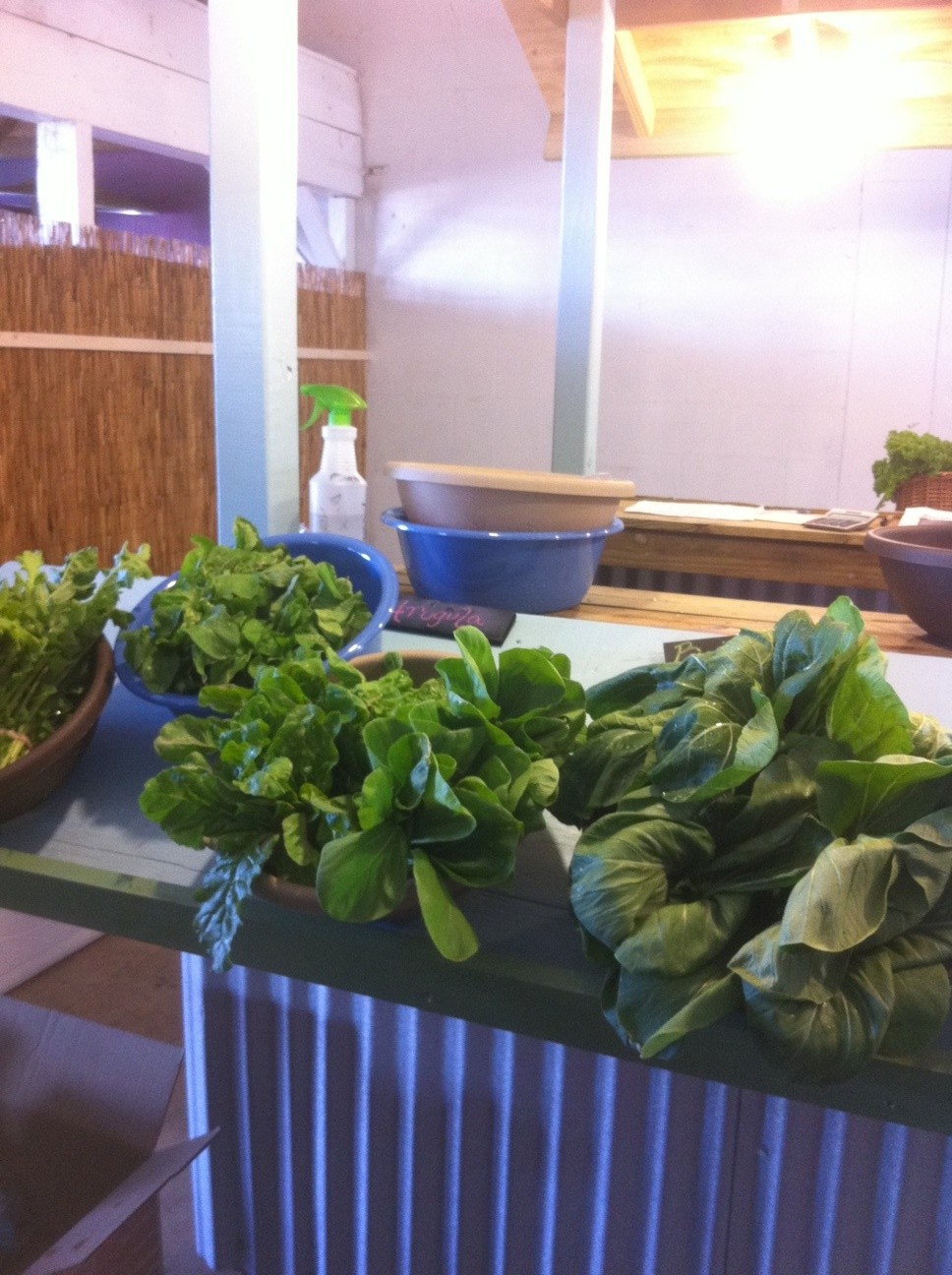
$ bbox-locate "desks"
[605,496,888,590]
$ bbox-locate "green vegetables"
[113,516,378,701]
[871,429,952,508]
[137,599,952,1088]
[0,535,154,772]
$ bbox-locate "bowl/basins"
[382,460,639,534]
[0,632,115,823]
[866,523,952,643]
[381,506,625,614]
[112,531,401,721]
